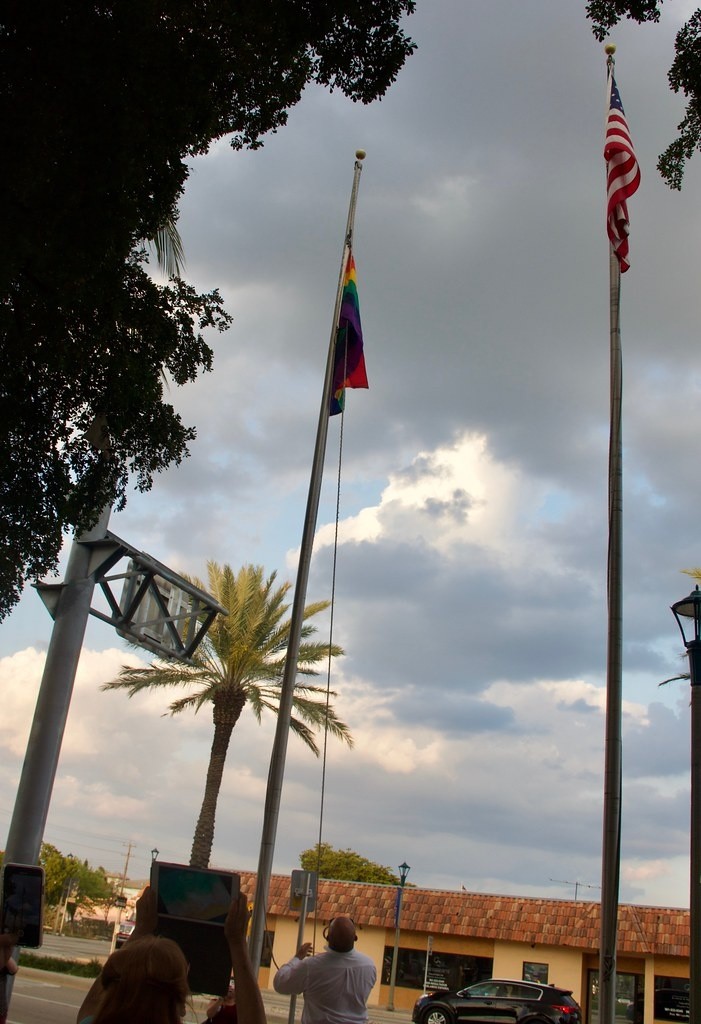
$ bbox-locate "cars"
[382,956,404,983]
[625,989,689,1023]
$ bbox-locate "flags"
[330,170,369,418]
[603,56,643,274]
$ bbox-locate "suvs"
[411,978,583,1024]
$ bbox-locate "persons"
[76,884,269,1024]
[273,916,378,1024]
[0,934,19,1024]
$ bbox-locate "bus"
[115,919,136,946]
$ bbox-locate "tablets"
[151,862,240,927]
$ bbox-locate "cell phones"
[2,863,45,949]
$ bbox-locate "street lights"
[53,852,74,932]
[150,847,159,864]
[387,861,412,1010]
[669,585,701,1022]
